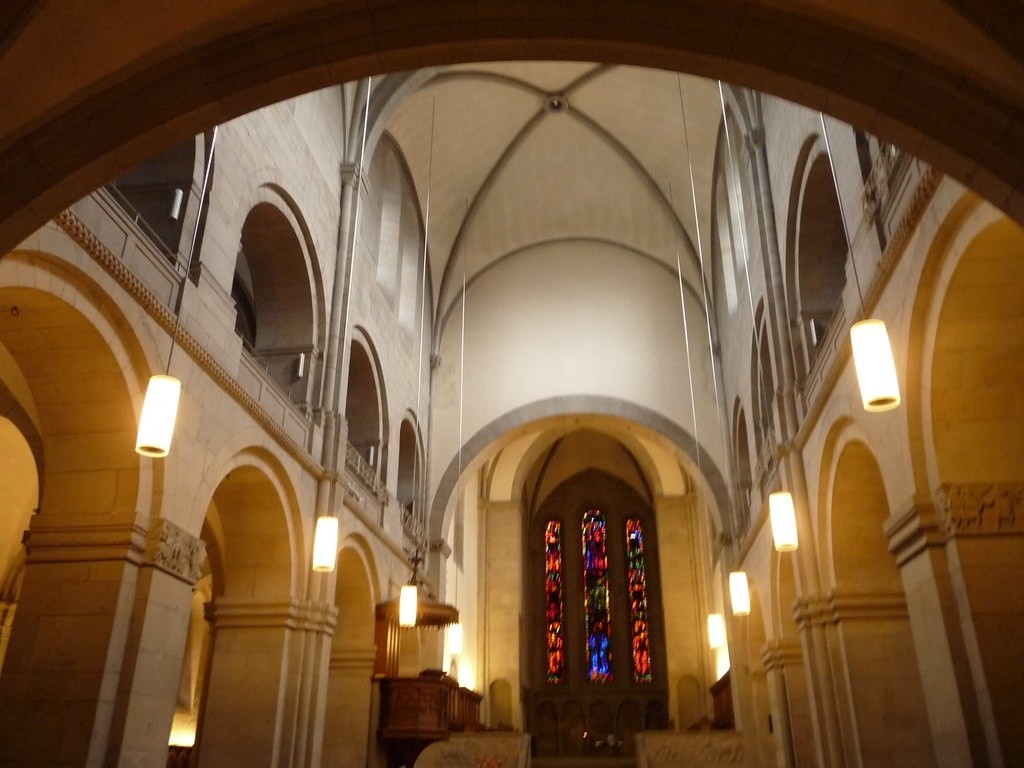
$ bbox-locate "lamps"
[312,75,372,573]
[820,113,901,412]
[135,125,219,458]
[718,81,798,552]
[399,96,435,627]
[677,72,752,617]
